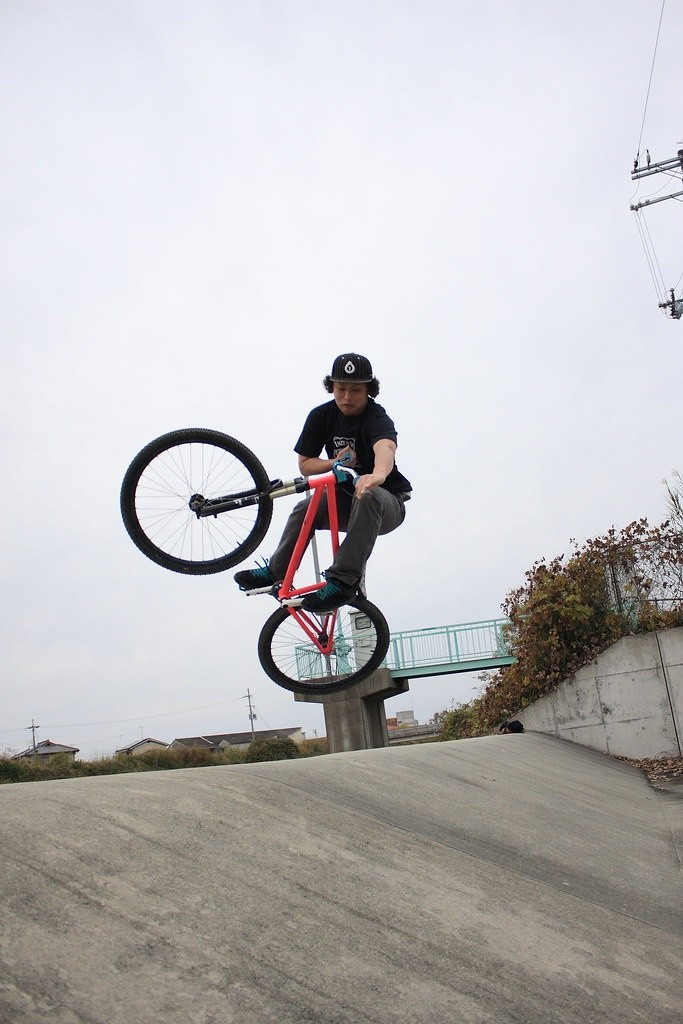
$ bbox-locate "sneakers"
[234,564,282,590]
[301,580,357,612]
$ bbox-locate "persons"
[233,353,414,613]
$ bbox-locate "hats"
[330,354,372,385]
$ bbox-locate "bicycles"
[117,426,391,695]
